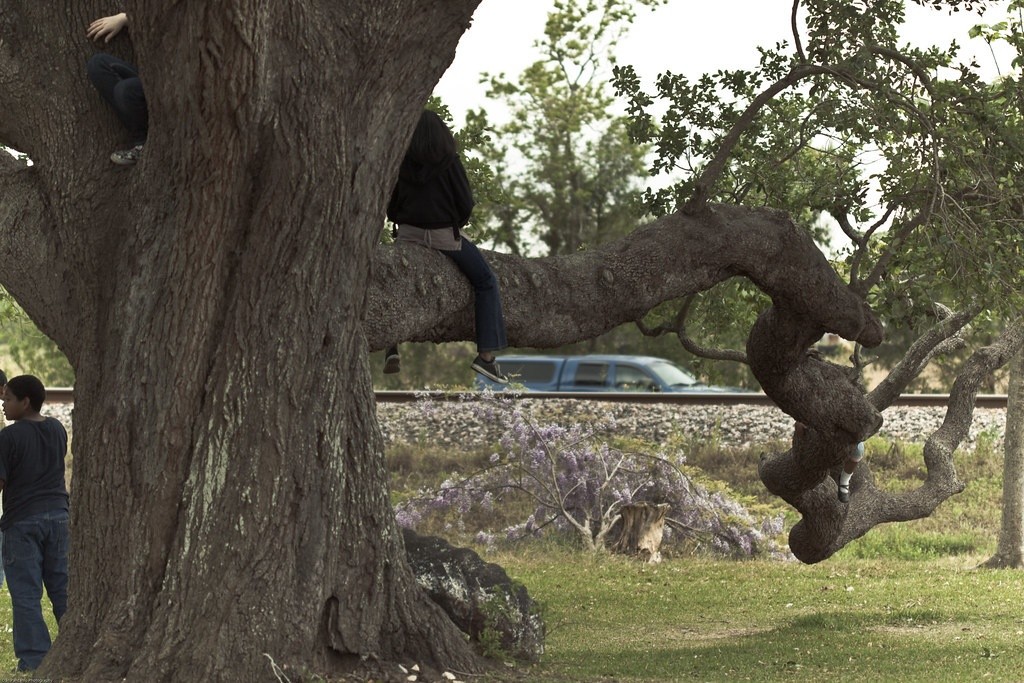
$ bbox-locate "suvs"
[473,353,753,395]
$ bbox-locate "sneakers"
[383,348,400,374]
[111,144,143,165]
[471,357,508,383]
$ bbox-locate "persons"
[382,110,509,384]
[0,369,15,429]
[0,375,70,673]
